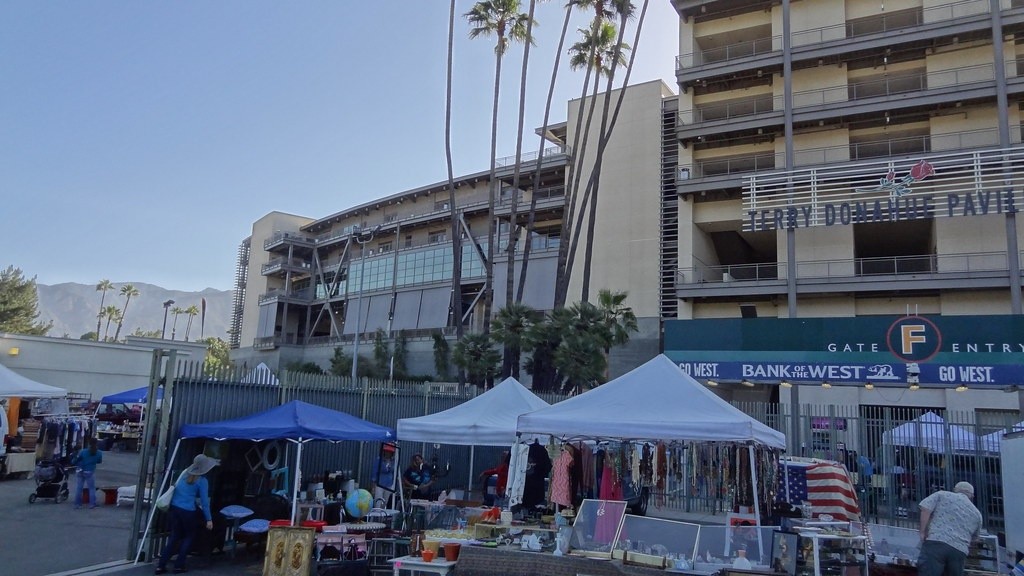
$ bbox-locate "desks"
[387,528,792,576]
[291,500,324,526]
[96,431,123,449]
[336,509,401,563]
[226,515,246,561]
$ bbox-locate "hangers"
[561,439,567,451]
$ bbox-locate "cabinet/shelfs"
[963,535,1000,575]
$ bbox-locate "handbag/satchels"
[156,469,187,511]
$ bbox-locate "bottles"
[732,549,752,570]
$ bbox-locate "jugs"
[521,533,543,550]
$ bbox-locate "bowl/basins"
[269,518,295,526]
[300,520,326,532]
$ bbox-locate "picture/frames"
[770,529,799,576]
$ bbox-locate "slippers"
[155,567,168,575]
[173,568,189,574]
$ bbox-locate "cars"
[484,468,652,517]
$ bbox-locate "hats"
[384,443,395,453]
[186,454,216,475]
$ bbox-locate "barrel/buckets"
[444,542,461,561]
[322,526,347,535]
[421,550,434,562]
[422,539,441,559]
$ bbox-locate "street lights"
[161,300,175,339]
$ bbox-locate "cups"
[801,501,812,520]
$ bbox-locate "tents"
[0,364,70,398]
[881,410,1024,504]
[134,353,788,567]
[93,385,173,452]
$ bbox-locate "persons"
[404,444,434,498]
[850,452,874,514]
[370,442,401,510]
[917,481,983,576]
[479,451,512,509]
[71,438,102,509]
[154,453,213,575]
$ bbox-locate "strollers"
[29,454,78,504]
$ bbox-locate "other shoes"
[89,505,95,509]
[74,505,80,509]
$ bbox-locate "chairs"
[232,493,290,568]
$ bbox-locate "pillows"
[218,504,254,517]
[239,519,270,533]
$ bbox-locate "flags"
[779,457,860,525]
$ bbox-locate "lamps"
[8,347,19,355]
